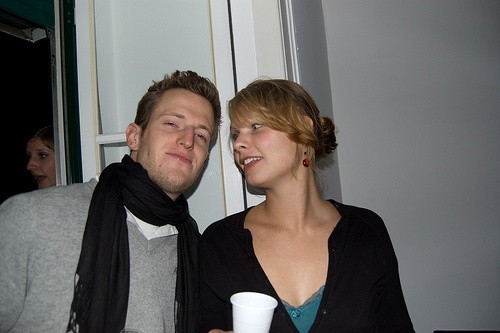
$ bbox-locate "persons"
[198,76,415,333]
[0,70,224,333]
[26,124,56,188]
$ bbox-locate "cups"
[230,292,278,333]
